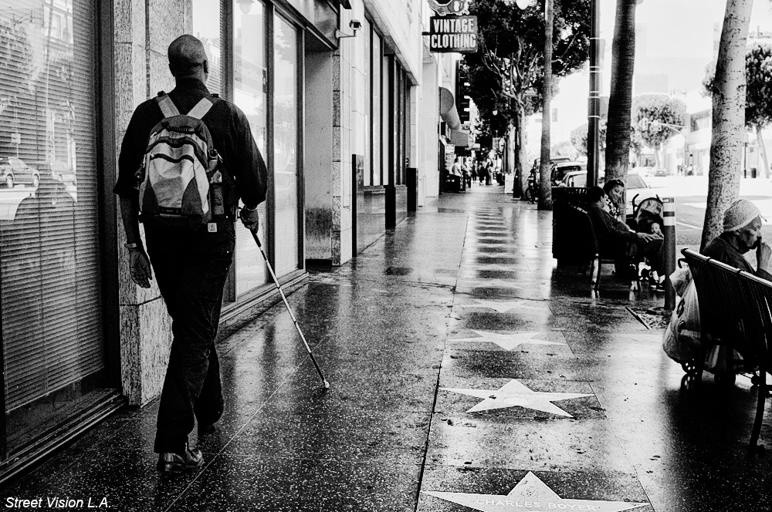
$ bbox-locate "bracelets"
[123,239,145,250]
[246,208,257,211]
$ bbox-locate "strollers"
[630,192,668,297]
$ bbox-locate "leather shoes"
[155,444,204,475]
[196,401,223,431]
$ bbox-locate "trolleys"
[674,255,754,377]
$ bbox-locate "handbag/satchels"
[135,89,228,222]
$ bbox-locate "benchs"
[679,245,772,462]
[570,202,644,296]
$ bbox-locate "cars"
[0,155,41,191]
[523,154,661,223]
[51,161,76,186]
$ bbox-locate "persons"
[649,221,664,238]
[111,34,270,475]
[700,199,772,282]
[452,155,494,189]
[587,185,667,294]
[602,179,627,225]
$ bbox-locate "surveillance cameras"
[349,19,361,31]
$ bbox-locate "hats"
[721,199,761,233]
